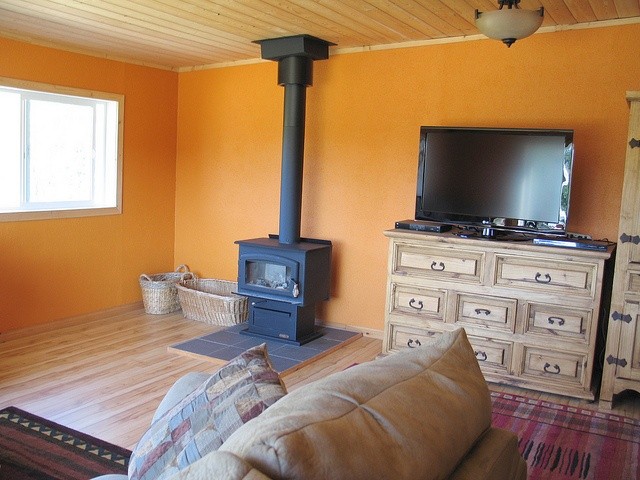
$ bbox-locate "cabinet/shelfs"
[599,91,640,410]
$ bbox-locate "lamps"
[474,0,545,48]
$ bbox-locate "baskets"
[175,272,249,327]
[140,265,192,314]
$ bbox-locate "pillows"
[126,342,288,479]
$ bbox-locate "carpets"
[487,389,640,480]
[0,405,132,480]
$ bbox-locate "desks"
[384,229,617,402]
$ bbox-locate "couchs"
[88,327,527,480]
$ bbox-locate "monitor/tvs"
[414,125,575,242]
